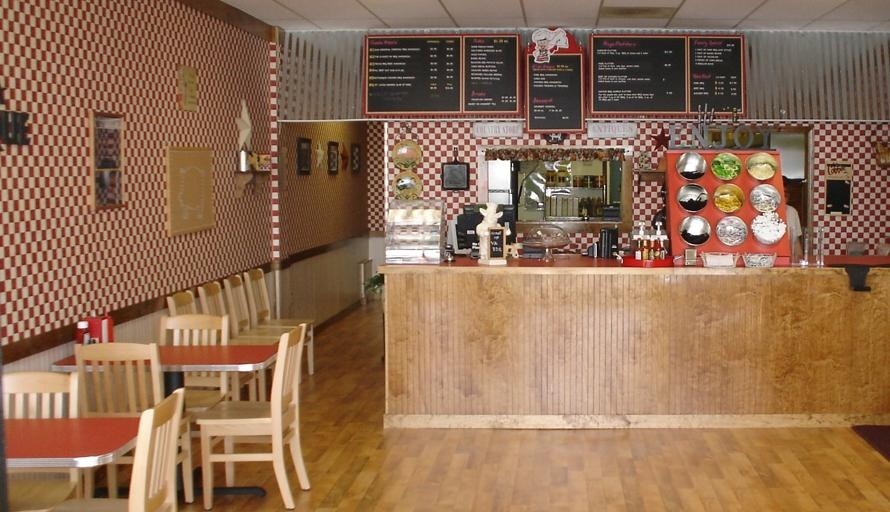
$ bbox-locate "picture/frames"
[296,136,313,175]
[439,162,470,190]
[349,143,362,174]
[327,141,339,174]
[87,108,128,209]
[488,227,506,260]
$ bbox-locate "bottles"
[579,196,604,217]
[545,169,567,186]
[75,321,92,344]
[634,238,666,261]
[572,175,603,188]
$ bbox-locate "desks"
[52,346,280,404]
[2,417,189,511]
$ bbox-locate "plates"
[391,140,423,200]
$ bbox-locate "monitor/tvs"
[463,205,517,246]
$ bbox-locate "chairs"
[166,290,256,401]
[194,322,309,511]
[242,268,312,375]
[224,275,297,376]
[2,370,82,510]
[197,282,280,401]
[44,387,186,511]
[73,343,196,503]
[156,313,237,486]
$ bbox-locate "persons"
[783,176,804,263]
